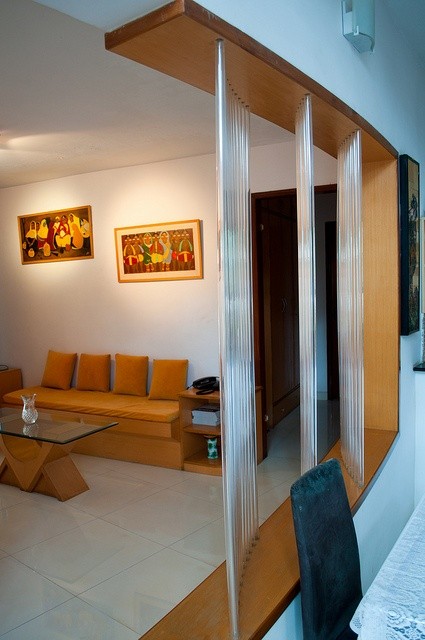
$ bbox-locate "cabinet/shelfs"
[0,365,23,407]
[255,201,300,427]
[180,379,266,476]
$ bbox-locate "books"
[191,403,221,426]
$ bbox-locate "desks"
[346,487,425,640]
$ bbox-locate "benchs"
[4,386,183,471]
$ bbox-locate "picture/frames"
[14,203,94,265]
[112,218,203,283]
[400,152,421,336]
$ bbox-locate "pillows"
[148,359,190,400]
[112,352,149,397]
[40,350,78,390]
[77,353,111,392]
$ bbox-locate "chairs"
[286,455,363,640]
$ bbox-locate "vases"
[21,392,39,425]
